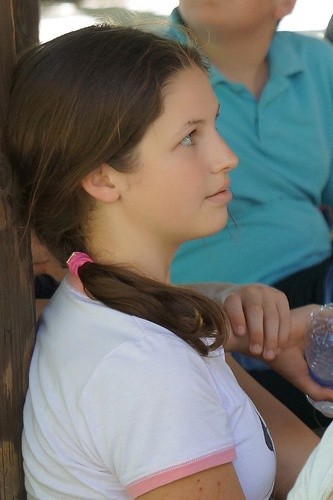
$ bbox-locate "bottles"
[305,302,333,418]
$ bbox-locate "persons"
[156,0,333,436]
[0,23,333,500]
[27,229,333,402]
